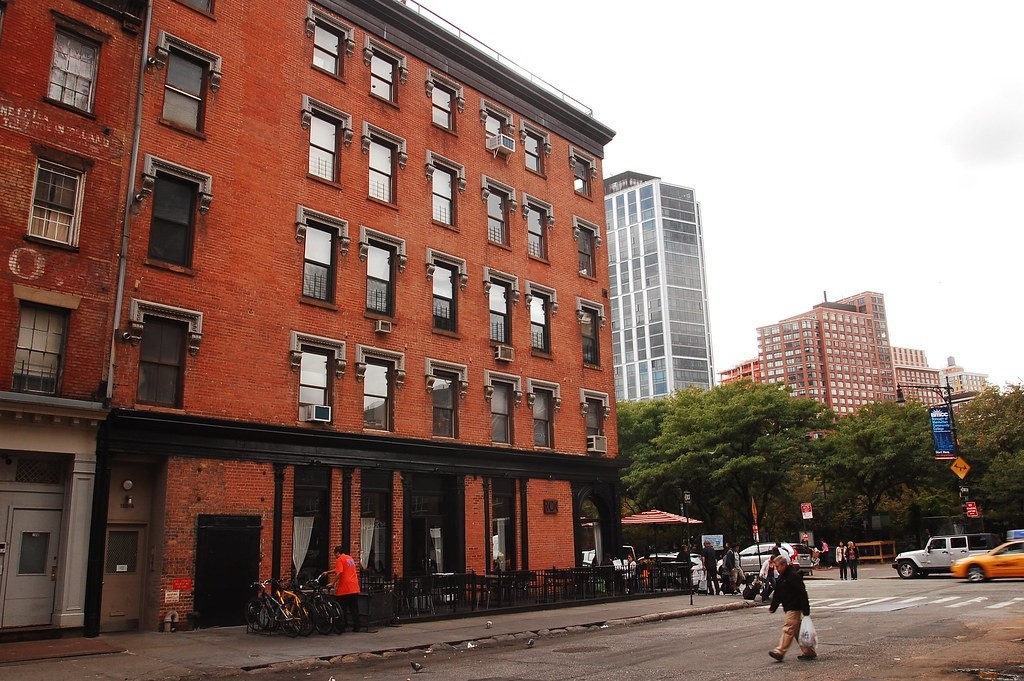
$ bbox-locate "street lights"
[894,375,970,533]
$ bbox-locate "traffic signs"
[959,486,969,497]
[966,502,979,518]
[683,491,692,504]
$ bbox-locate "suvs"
[715,541,814,584]
[581,545,637,582]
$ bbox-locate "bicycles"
[245,572,346,638]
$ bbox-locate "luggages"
[697,570,708,596]
[743,575,764,600]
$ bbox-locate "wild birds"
[410,661,425,673]
[329,675,336,681]
[486,619,493,628]
[527,639,534,648]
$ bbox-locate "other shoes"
[797,654,817,660]
[763,599,767,603]
[768,651,783,661]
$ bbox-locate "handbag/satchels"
[798,616,818,649]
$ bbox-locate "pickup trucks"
[890,534,1009,579]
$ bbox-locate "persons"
[923,528,932,550]
[622,554,636,594]
[801,534,808,547]
[322,545,361,634]
[701,539,720,595]
[372,560,384,572]
[726,541,745,594]
[638,552,655,592]
[676,545,692,591]
[759,555,780,603]
[768,555,818,661]
[813,537,832,570]
[836,540,848,580]
[845,541,859,580]
[743,574,760,600]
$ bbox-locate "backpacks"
[722,549,733,569]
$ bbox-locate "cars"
[950,528,1024,584]
[637,552,706,590]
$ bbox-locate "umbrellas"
[582,507,703,592]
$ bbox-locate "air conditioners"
[586,435,608,453]
[495,346,515,361]
[306,405,333,422]
[375,320,392,333]
[489,134,516,160]
